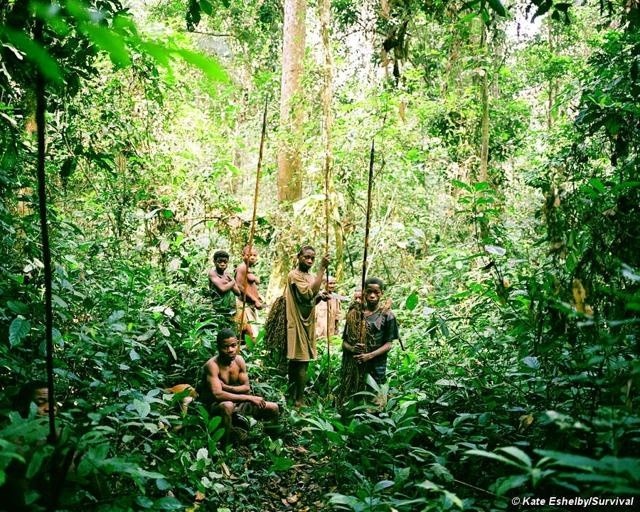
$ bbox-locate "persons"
[285,244,334,409]
[314,274,341,339]
[207,249,256,346]
[352,287,366,306]
[164,382,202,433]
[339,277,399,414]
[17,379,57,423]
[236,245,271,347]
[198,328,280,444]
